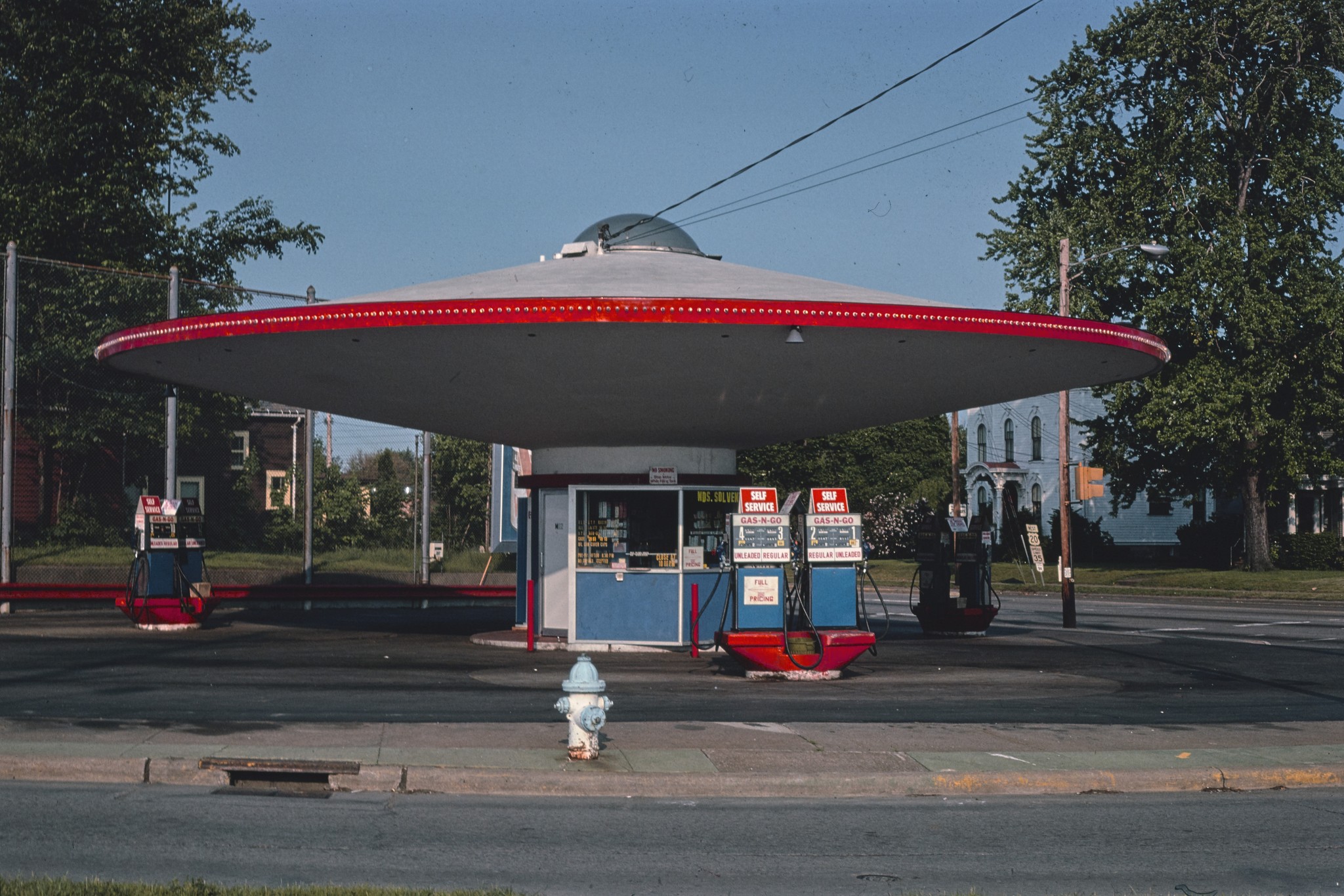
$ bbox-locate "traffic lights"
[1074,466,1104,501]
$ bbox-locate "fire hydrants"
[553,652,614,760]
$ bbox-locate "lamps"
[785,325,803,344]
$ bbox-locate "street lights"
[1056,239,1168,630]
